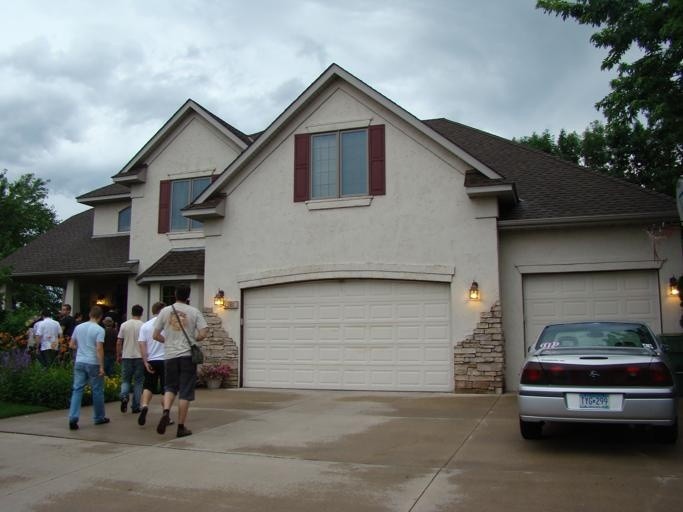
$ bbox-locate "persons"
[68,305,109,430]
[114,304,146,414]
[150,282,208,437]
[137,302,174,426]
[25,304,127,376]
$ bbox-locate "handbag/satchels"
[192,344,203,365]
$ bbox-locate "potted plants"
[199,360,232,389]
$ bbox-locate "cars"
[515,320,678,449]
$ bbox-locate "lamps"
[214,288,224,306]
[467,281,480,301]
[668,276,680,296]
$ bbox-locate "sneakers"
[94,418,110,424]
[70,417,78,430]
[157,410,192,437]
[121,398,148,425]
